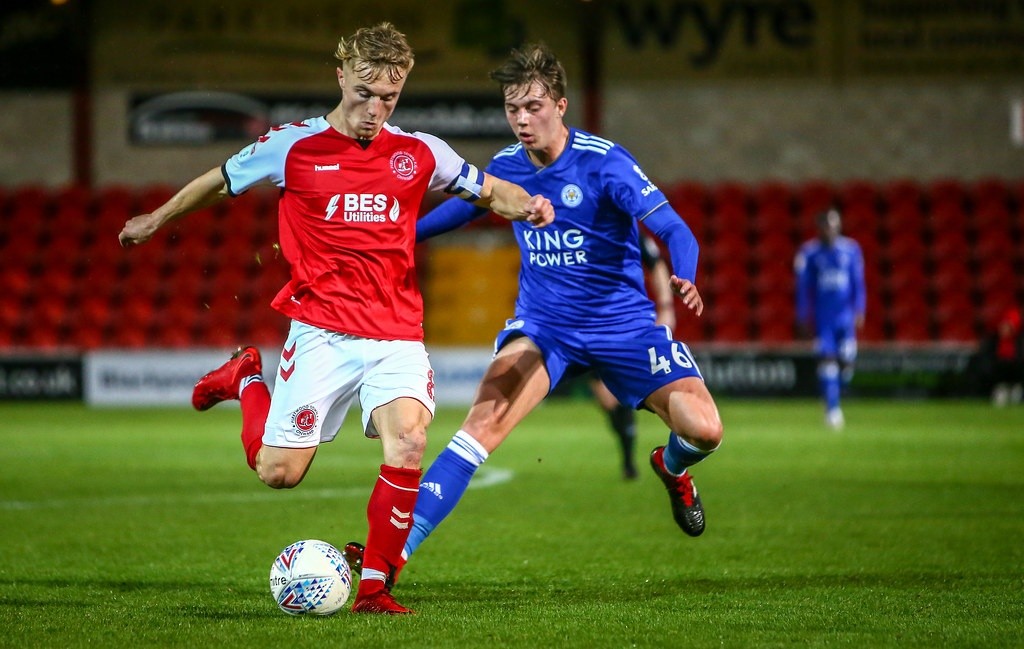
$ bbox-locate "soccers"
[268,538,353,618]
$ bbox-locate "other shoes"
[825,406,847,429]
[622,453,636,478]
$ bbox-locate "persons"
[342,41,723,593]
[793,206,866,429]
[577,226,677,479]
[119,21,554,614]
[561,229,676,479]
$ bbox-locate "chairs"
[0,174,1024,352]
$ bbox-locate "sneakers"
[343,541,401,593]
[649,445,706,538]
[352,587,417,615]
[191,344,263,412]
